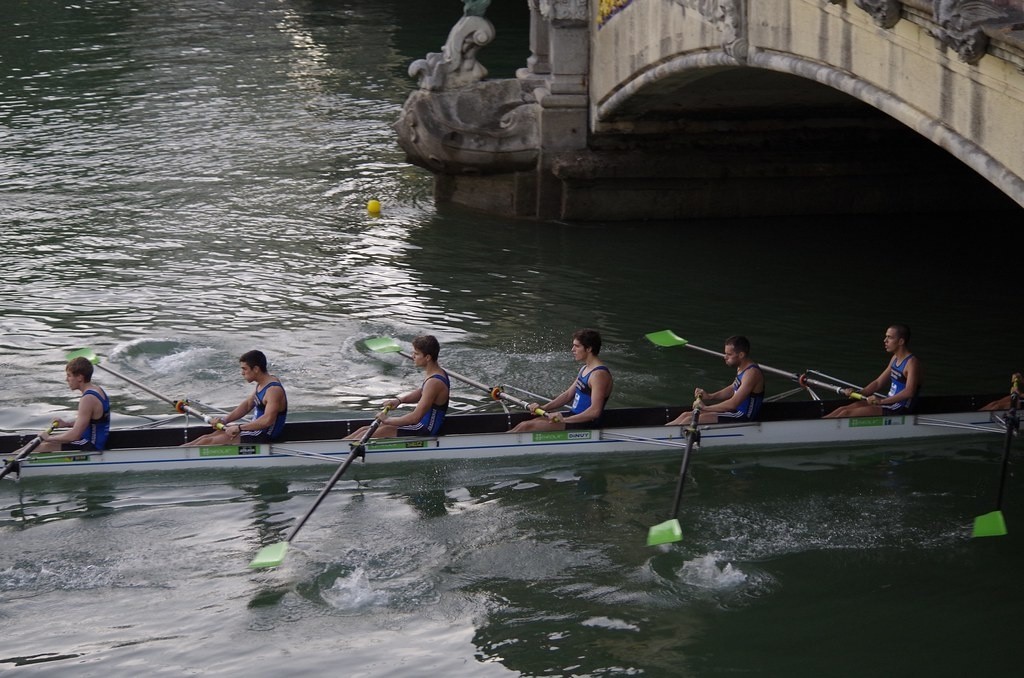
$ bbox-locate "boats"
[0,396,1024,485]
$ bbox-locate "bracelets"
[398,399,401,404]
[238,423,243,432]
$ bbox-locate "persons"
[342,335,450,440]
[821,323,921,419]
[180,349,288,446]
[506,330,613,434]
[978,373,1024,410]
[12,357,111,454]
[665,335,765,426]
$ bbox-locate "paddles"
[364,336,560,424]
[66,347,229,432]
[645,329,869,402]
[246,405,392,569]
[0,421,60,482]
[971,381,1018,539]
[645,392,705,548]
